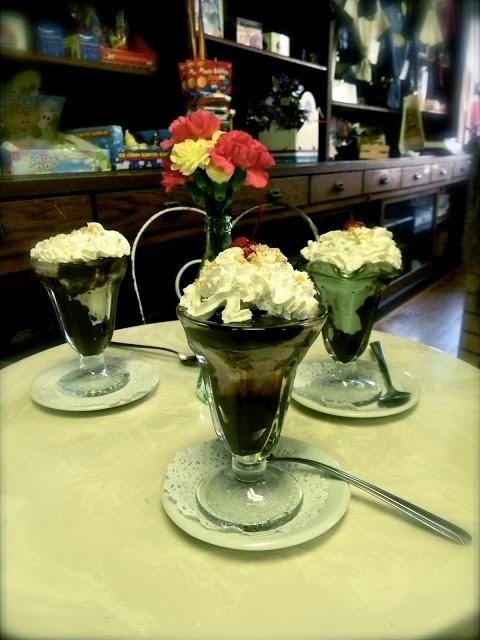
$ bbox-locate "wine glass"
[31,254,132,396]
[307,262,404,406]
[176,303,328,532]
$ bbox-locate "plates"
[159,433,352,551]
[27,354,161,413]
[292,360,422,420]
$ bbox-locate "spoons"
[105,341,198,365]
[371,341,411,405]
